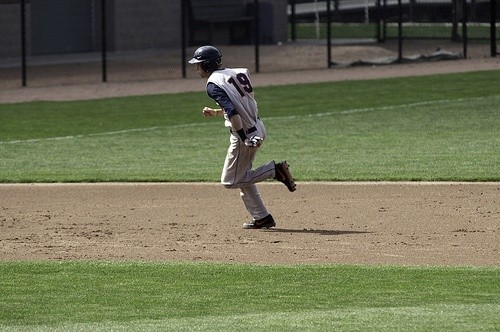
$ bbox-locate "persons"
[188,46,297,228]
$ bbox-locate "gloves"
[243,135,264,147]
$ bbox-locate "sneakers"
[242,214,276,229]
[276,160,297,192]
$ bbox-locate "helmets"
[188,45,223,64]
